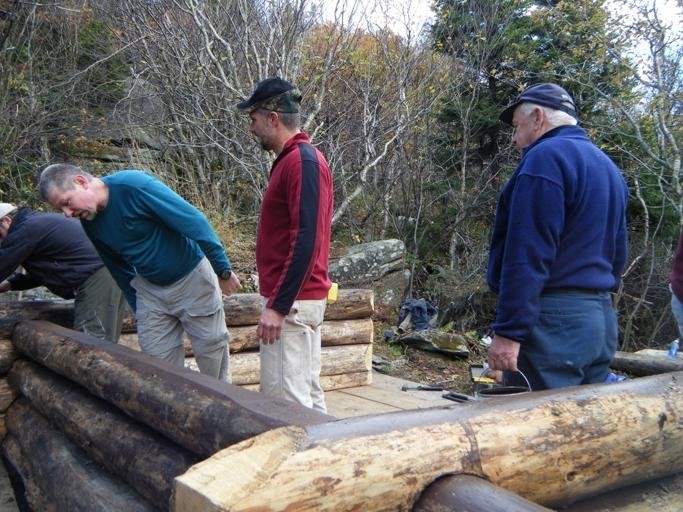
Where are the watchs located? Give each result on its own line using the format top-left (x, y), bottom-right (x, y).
top-left (217, 270), bottom-right (231, 280)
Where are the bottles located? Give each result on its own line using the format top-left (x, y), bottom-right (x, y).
top-left (667, 339), bottom-right (680, 359)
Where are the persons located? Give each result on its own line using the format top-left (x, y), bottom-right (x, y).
top-left (672, 224), bottom-right (683, 338)
top-left (237, 77), bottom-right (333, 414)
top-left (0, 203), bottom-right (126, 343)
top-left (40, 164), bottom-right (242, 383)
top-left (487, 84), bottom-right (628, 391)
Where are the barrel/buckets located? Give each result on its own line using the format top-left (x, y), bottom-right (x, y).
top-left (469, 362), bottom-right (534, 397)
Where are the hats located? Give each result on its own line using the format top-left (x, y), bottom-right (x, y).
top-left (0, 203), bottom-right (18, 219)
top-left (237, 78), bottom-right (303, 115)
top-left (498, 82), bottom-right (577, 127)
